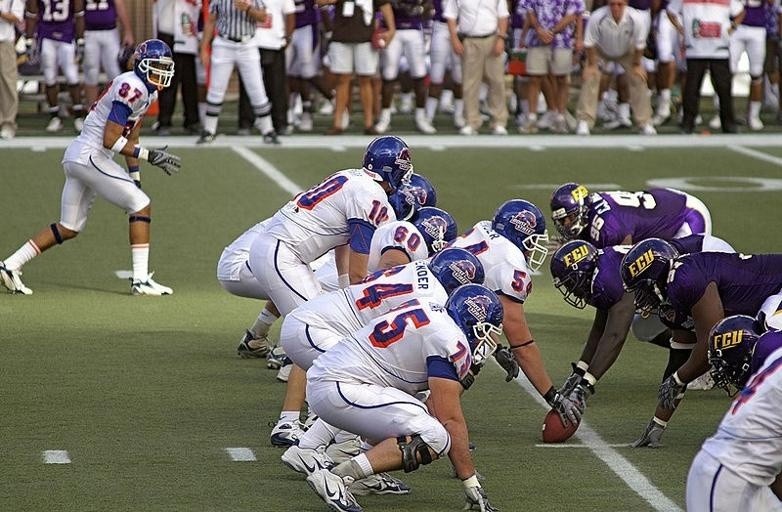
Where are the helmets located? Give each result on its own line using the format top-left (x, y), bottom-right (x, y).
top-left (133, 38), bottom-right (176, 91)
top-left (363, 136), bottom-right (503, 366)
top-left (550, 240), bottom-right (598, 309)
top-left (708, 315), bottom-right (762, 398)
top-left (619, 238), bottom-right (679, 318)
top-left (551, 183), bottom-right (592, 242)
top-left (492, 199), bottom-right (549, 272)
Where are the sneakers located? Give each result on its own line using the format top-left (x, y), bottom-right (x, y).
top-left (254, 118), bottom-right (281, 143)
top-left (268, 414), bottom-right (411, 512)
top-left (159, 122), bottom-right (214, 143)
top-left (0, 260), bottom-right (33, 295)
top-left (238, 329), bottom-right (293, 383)
top-left (129, 271), bottom-right (173, 296)
top-left (46, 116), bottom-right (85, 134)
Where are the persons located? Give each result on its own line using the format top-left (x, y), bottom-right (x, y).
top-left (0, 0), bottom-right (27, 141)
top-left (30, 0), bottom-right (87, 135)
top-left (151, 0), bottom-right (781, 145)
top-left (218, 135), bottom-right (580, 510)
top-left (0, 37), bottom-right (183, 297)
top-left (81, 0), bottom-right (135, 113)
top-left (550, 183), bottom-right (782, 511)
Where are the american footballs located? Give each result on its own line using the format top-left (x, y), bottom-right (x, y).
top-left (543, 409), bottom-right (582, 442)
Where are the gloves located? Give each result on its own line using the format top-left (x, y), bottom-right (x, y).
top-left (492, 343), bottom-right (519, 382)
top-left (148, 145), bottom-right (181, 175)
top-left (543, 362), bottom-right (595, 427)
top-left (657, 374), bottom-right (682, 410)
top-left (632, 421), bottom-right (667, 449)
top-left (461, 473), bottom-right (499, 512)
